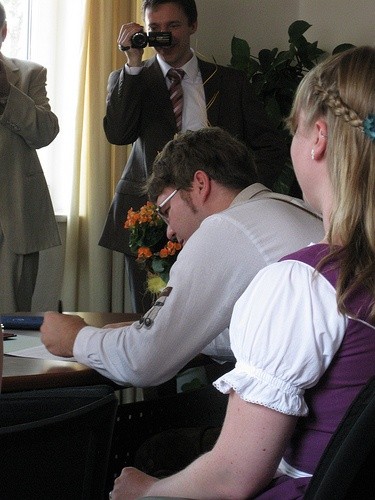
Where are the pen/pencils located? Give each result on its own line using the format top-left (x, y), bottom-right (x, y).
top-left (58, 299), bottom-right (63, 314)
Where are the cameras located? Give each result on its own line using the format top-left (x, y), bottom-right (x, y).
top-left (130, 26), bottom-right (173, 48)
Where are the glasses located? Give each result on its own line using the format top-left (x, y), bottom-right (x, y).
top-left (153, 186), bottom-right (179, 225)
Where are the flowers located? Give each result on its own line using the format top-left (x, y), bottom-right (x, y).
top-left (124, 200), bottom-right (182, 293)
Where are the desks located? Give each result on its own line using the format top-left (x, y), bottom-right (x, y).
top-left (0, 311), bottom-right (207, 500)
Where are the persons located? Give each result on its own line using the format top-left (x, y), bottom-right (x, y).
top-left (41, 124), bottom-right (329, 388)
top-left (98, 0), bottom-right (267, 313)
top-left (0, 2), bottom-right (61, 311)
top-left (111, 45), bottom-right (375, 500)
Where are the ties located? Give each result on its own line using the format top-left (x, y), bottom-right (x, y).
top-left (165, 68), bottom-right (185, 139)
